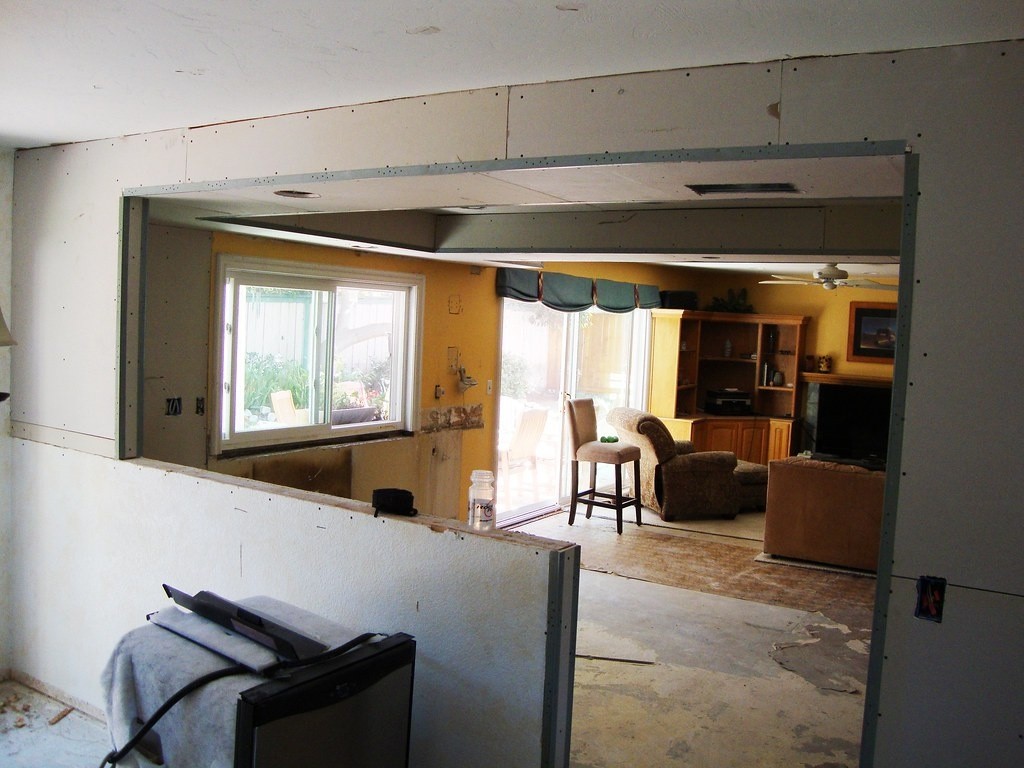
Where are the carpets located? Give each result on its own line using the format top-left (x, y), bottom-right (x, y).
top-left (518, 517), bottom-right (878, 613)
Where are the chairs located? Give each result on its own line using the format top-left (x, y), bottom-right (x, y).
top-left (564, 397), bottom-right (642, 536)
top-left (499, 408), bottom-right (549, 511)
top-left (270, 390), bottom-right (298, 424)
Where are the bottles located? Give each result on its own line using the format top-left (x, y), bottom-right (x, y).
top-left (723, 338), bottom-right (732, 357)
top-left (804, 355), bottom-right (817, 371)
top-left (468, 469), bottom-right (494, 532)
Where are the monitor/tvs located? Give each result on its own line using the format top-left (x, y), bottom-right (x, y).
top-left (814, 383), bottom-right (892, 466)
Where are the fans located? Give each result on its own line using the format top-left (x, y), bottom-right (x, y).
top-left (757, 264), bottom-right (899, 290)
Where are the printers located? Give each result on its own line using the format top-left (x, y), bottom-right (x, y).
top-left (704, 388), bottom-right (753, 416)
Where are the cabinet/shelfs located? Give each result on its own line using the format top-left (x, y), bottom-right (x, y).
top-left (647, 309), bottom-right (811, 465)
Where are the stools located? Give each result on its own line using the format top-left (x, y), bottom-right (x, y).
top-left (733, 459), bottom-right (768, 514)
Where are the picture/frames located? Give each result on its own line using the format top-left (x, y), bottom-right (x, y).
top-left (846, 301), bottom-right (898, 364)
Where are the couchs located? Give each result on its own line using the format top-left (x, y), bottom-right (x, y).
top-left (627, 415), bottom-right (739, 522)
top-left (763, 452), bottom-right (885, 573)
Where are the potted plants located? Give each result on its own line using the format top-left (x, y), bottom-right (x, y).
top-left (330, 392), bottom-right (376, 424)
